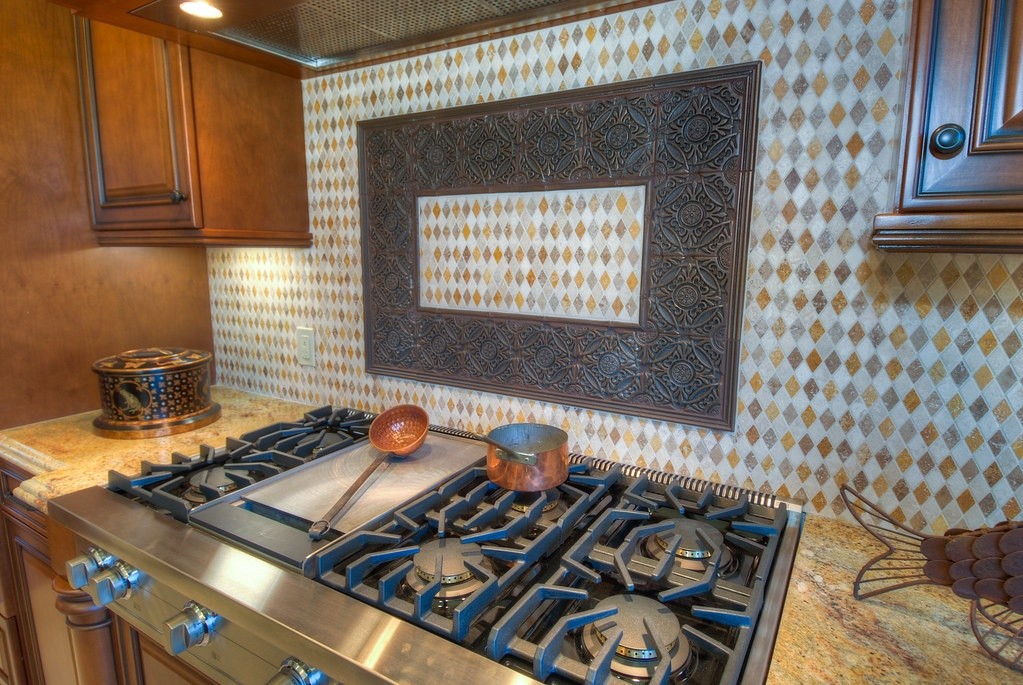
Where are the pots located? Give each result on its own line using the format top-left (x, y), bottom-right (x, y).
top-left (474, 423), bottom-right (569, 491)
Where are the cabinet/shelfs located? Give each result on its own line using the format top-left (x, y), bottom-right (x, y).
top-left (869, 0), bottom-right (1023, 254)
top-left (72, 15), bottom-right (313, 249)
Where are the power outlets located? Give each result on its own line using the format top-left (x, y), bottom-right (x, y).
top-left (296, 326), bottom-right (315, 366)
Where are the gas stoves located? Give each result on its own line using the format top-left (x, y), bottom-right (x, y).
top-left (47, 405), bottom-right (803, 685)
